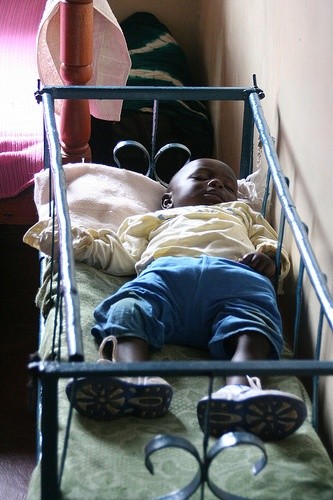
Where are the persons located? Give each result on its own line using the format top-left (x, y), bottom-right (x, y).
top-left (23, 159), bottom-right (307, 442)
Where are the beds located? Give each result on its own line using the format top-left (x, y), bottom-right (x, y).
top-left (0, 0), bottom-right (94, 228)
top-left (28, 76), bottom-right (333, 500)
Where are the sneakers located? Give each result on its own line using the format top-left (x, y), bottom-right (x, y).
top-left (65, 358), bottom-right (174, 422)
top-left (197, 375), bottom-right (307, 441)
